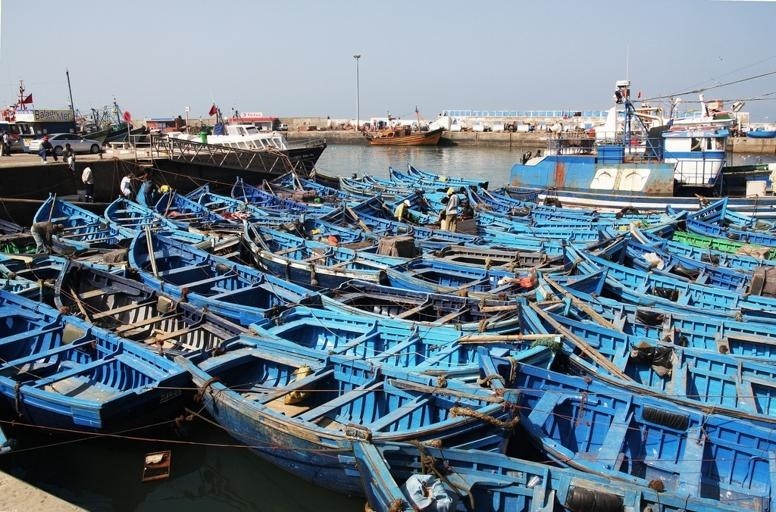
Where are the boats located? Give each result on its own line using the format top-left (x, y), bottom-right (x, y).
top-left (0, 165), bottom-right (776, 512)
top-left (747, 129), bottom-right (775, 138)
top-left (81, 123), bottom-right (151, 146)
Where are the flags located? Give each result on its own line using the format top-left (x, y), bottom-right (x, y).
top-left (209, 105), bottom-right (217, 116)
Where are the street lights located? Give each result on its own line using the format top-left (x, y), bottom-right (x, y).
top-left (352, 54), bottom-right (361, 131)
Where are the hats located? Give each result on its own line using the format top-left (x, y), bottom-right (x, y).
top-left (44, 136), bottom-right (48, 141)
top-left (404, 200), bottom-right (411, 207)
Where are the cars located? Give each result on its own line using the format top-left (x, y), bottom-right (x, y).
top-left (29, 132), bottom-right (102, 157)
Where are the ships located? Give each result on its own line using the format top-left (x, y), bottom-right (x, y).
top-left (369, 126), bottom-right (443, 146)
top-left (511, 109), bottom-right (776, 221)
top-left (163, 125), bottom-right (326, 174)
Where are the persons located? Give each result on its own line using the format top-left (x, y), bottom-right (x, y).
top-left (120, 174), bottom-right (132, 200)
top-left (62, 143), bottom-right (75, 174)
top-left (81, 163), bottom-right (94, 201)
top-left (446, 187), bottom-right (459, 232)
top-left (39, 136), bottom-right (58, 163)
top-left (0, 132), bottom-right (13, 156)
top-left (135, 172), bottom-right (151, 207)
